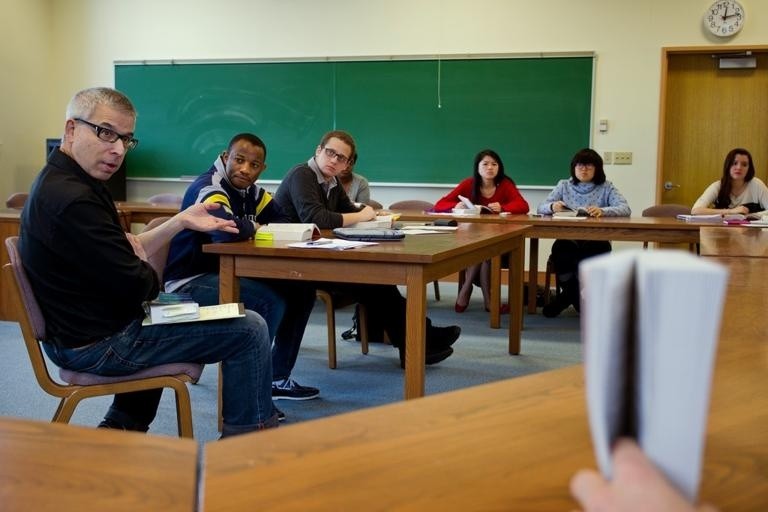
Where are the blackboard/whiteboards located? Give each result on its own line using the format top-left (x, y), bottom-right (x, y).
top-left (114, 55), bottom-right (593, 188)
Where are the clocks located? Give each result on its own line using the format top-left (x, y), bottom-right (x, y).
top-left (704, 0), bottom-right (745, 37)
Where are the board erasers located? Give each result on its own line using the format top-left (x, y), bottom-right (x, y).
top-left (182, 176), bottom-right (199, 181)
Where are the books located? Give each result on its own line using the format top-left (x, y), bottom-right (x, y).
top-left (579, 249), bottom-right (731, 501)
top-left (677, 213), bottom-right (722, 223)
top-left (723, 210), bottom-right (768, 221)
top-left (255, 223), bottom-right (321, 241)
top-left (148, 291), bottom-right (200, 325)
top-left (346, 211), bottom-right (404, 230)
top-left (551, 202), bottom-right (590, 218)
top-left (255, 240), bottom-right (310, 248)
top-left (452, 195), bottom-right (491, 216)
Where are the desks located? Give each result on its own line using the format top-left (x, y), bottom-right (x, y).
top-left (0, 202), bottom-right (768, 512)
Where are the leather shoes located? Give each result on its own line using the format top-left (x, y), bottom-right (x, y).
top-left (455, 285), bottom-right (473, 313)
top-left (484, 303), bottom-right (511, 314)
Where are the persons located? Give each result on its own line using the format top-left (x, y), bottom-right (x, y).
top-left (16, 87), bottom-right (280, 443)
top-left (537, 147), bottom-right (631, 317)
top-left (690, 148), bottom-right (767, 215)
top-left (160, 133), bottom-right (321, 402)
top-left (337, 150), bottom-right (371, 206)
top-left (272, 132), bottom-right (461, 371)
top-left (432, 150), bottom-right (529, 314)
top-left (570, 435), bottom-right (717, 512)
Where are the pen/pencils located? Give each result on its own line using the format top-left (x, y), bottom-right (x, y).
top-left (307, 240), bottom-right (333, 245)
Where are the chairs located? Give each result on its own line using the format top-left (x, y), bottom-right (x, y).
top-left (145, 194), bottom-right (182, 225)
top-left (6, 193), bottom-right (28, 208)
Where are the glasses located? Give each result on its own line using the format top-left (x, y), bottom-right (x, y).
top-left (325, 148), bottom-right (348, 163)
top-left (73, 117), bottom-right (138, 151)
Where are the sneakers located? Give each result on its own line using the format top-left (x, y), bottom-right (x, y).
top-left (399, 317), bottom-right (461, 369)
top-left (271, 378), bottom-right (320, 401)
